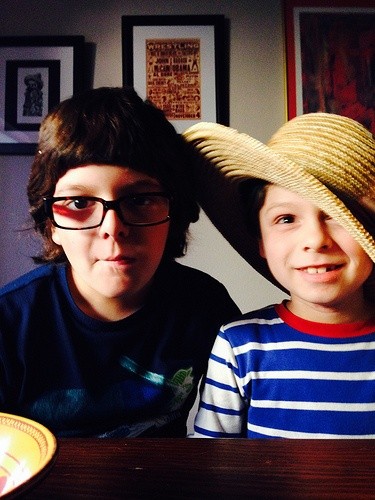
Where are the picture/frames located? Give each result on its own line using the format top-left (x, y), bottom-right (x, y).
top-left (120, 15), bottom-right (230, 138)
top-left (281, 0), bottom-right (375, 139)
top-left (0, 34), bottom-right (88, 155)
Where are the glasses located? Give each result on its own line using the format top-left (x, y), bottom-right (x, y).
top-left (43, 190), bottom-right (182, 231)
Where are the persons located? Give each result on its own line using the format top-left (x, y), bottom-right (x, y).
top-left (1, 87), bottom-right (242, 436)
top-left (180, 112), bottom-right (375, 440)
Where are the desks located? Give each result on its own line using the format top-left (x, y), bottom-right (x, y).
top-left (0, 439), bottom-right (375, 500)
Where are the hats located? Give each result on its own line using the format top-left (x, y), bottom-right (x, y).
top-left (182, 112), bottom-right (375, 297)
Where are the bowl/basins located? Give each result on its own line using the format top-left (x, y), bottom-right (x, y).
top-left (0, 413), bottom-right (59, 500)
